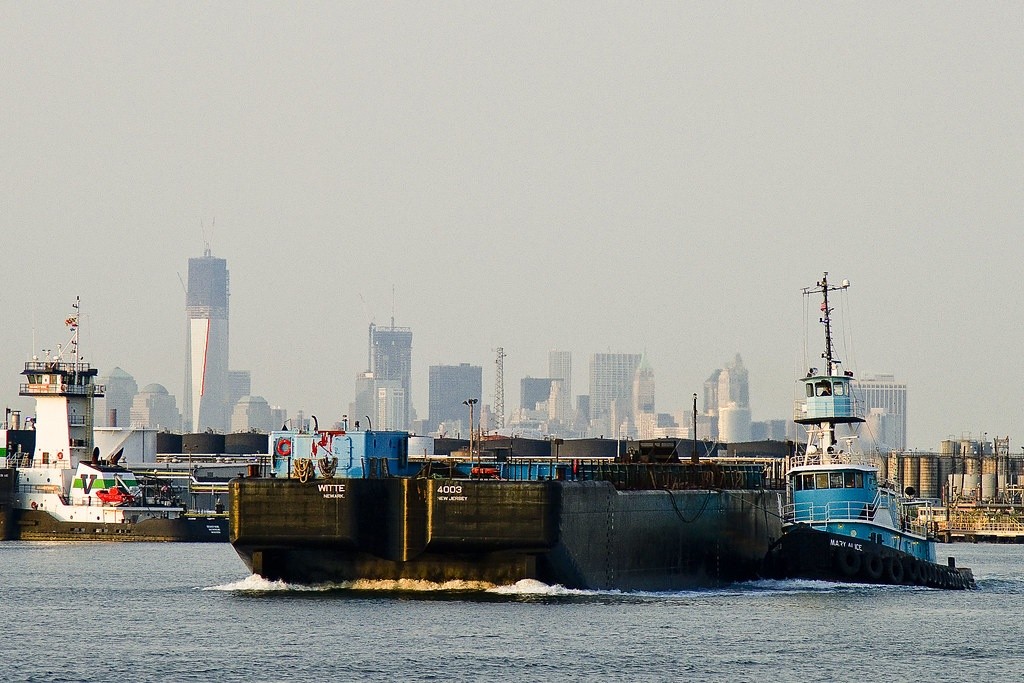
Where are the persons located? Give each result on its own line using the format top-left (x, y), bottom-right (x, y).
top-left (820, 386), bottom-right (832, 396)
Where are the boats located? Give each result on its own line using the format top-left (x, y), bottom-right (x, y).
top-left (0, 295), bottom-right (229, 544)
top-left (227, 410), bottom-right (788, 590)
top-left (95, 489), bottom-right (133, 503)
top-left (769, 270), bottom-right (975, 589)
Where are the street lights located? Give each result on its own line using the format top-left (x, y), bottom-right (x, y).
top-left (463, 398), bottom-right (478, 463)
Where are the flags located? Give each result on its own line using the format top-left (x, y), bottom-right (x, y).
top-left (65, 318), bottom-right (78, 331)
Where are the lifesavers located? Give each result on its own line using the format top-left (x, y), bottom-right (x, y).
top-left (905, 556), bottom-right (919, 582)
top-left (471, 466), bottom-right (499, 475)
top-left (886, 555), bottom-right (905, 585)
top-left (57, 452), bottom-right (63, 459)
top-left (837, 549), bottom-right (861, 574)
top-left (920, 560), bottom-right (967, 590)
top-left (31, 502), bottom-right (37, 509)
top-left (277, 440), bottom-right (293, 456)
top-left (864, 552), bottom-right (884, 580)
